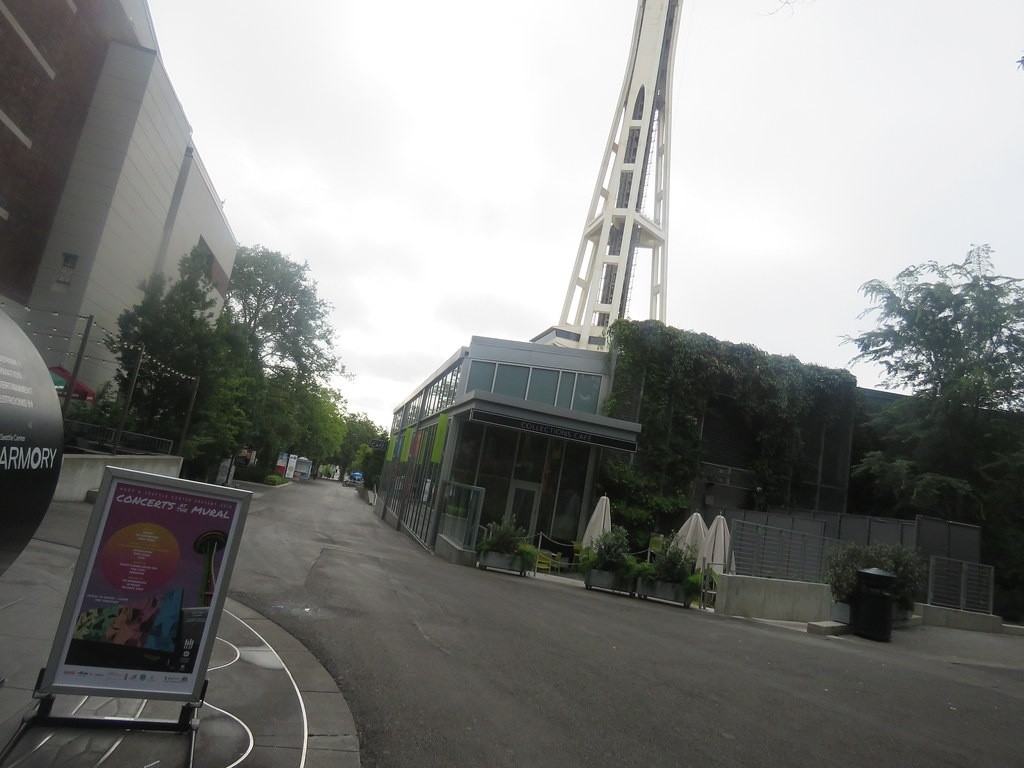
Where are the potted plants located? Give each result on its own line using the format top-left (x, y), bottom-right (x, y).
top-left (826, 542), bottom-right (927, 624)
top-left (478, 514), bottom-right (537, 577)
top-left (570, 524), bottom-right (718, 608)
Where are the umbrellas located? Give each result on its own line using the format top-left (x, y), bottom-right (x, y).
top-left (48, 362), bottom-right (95, 401)
top-left (667, 512), bottom-right (736, 576)
top-left (579, 493), bottom-right (612, 557)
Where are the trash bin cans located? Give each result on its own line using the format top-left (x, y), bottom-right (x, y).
top-left (849, 567), bottom-right (898, 641)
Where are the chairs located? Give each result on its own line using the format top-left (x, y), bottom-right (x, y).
top-left (537, 550), bottom-right (562, 575)
